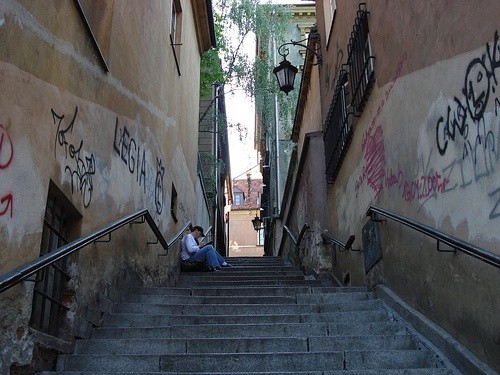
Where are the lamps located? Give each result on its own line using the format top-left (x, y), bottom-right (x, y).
top-left (272, 32), bottom-right (321, 96)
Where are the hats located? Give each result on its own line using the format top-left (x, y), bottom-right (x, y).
top-left (192, 225), bottom-right (206, 237)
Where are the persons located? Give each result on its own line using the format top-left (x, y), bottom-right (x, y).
top-left (181, 225), bottom-right (234, 272)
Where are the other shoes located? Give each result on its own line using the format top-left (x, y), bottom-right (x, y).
top-left (210, 267), bottom-right (220, 271)
top-left (221, 263), bottom-right (233, 268)
